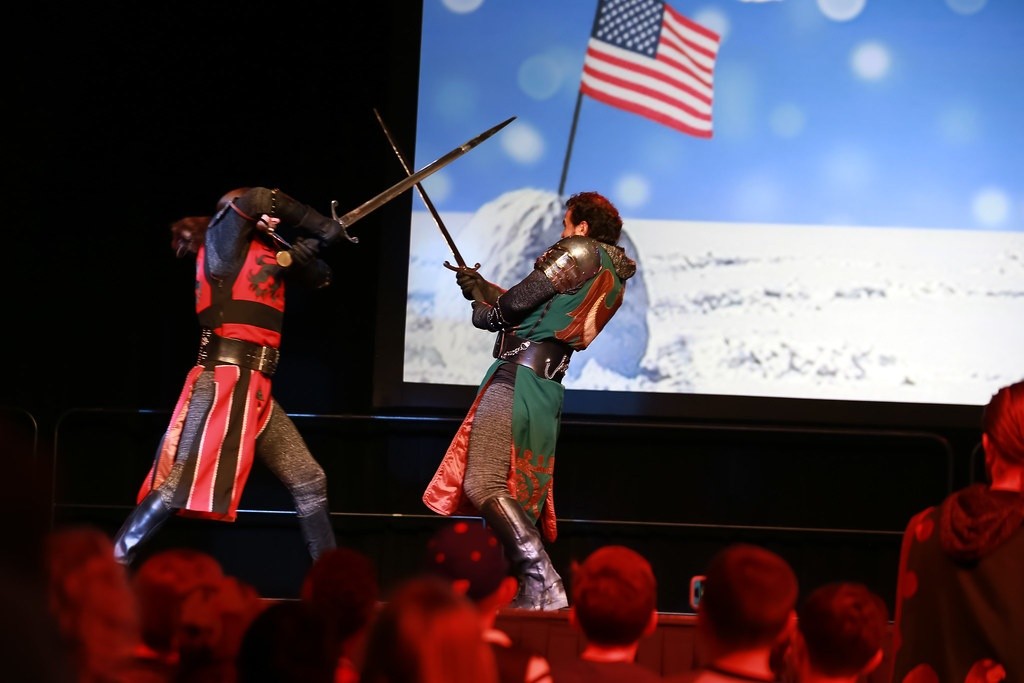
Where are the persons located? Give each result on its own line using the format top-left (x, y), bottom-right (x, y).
top-left (109, 187), bottom-right (382, 597)
top-left (777, 580), bottom-right (887, 683)
top-left (14, 519), bottom-right (553, 683)
top-left (424, 192), bottom-right (636, 612)
top-left (553, 544), bottom-right (668, 683)
top-left (888, 373), bottom-right (1023, 682)
top-left (672, 544), bottom-right (800, 683)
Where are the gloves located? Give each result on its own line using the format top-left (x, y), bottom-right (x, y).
top-left (289, 234), bottom-right (333, 293)
top-left (291, 204), bottom-right (347, 253)
top-left (456, 267), bottom-right (503, 307)
top-left (470, 301), bottom-right (499, 333)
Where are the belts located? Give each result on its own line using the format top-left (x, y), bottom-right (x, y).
top-left (491, 329), bottom-right (575, 385)
top-left (198, 332), bottom-right (281, 377)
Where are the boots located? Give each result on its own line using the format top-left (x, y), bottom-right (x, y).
top-left (479, 495), bottom-right (569, 612)
top-left (109, 489), bottom-right (176, 568)
top-left (295, 508), bottom-right (335, 566)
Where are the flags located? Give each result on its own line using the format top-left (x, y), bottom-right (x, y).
top-left (582, 2), bottom-right (722, 135)
